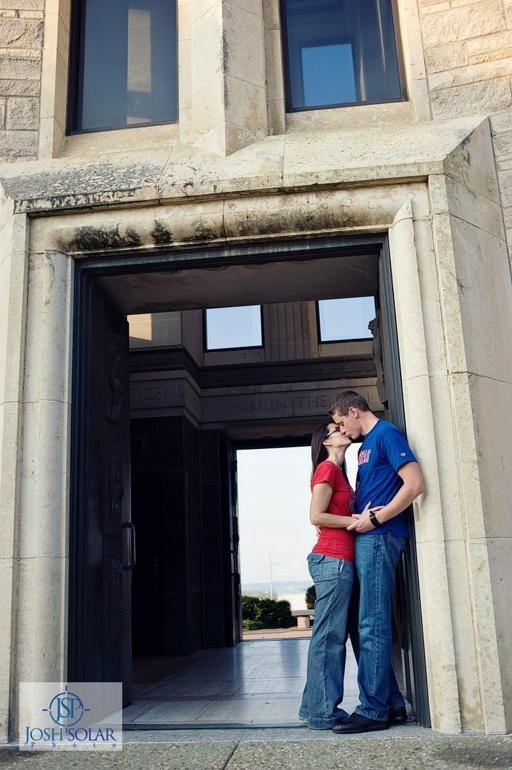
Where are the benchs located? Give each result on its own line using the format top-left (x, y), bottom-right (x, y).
top-left (291, 609), bottom-right (316, 629)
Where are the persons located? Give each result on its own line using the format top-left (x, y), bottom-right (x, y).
top-left (328, 390), bottom-right (425, 734)
top-left (298, 421), bottom-right (384, 731)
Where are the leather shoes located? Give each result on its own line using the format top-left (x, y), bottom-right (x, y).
top-left (332, 712), bottom-right (390, 734)
top-left (389, 708), bottom-right (407, 725)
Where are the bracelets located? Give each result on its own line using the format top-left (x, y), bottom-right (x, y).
top-left (369, 510), bottom-right (382, 528)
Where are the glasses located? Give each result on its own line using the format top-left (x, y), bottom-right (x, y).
top-left (327, 427), bottom-right (340, 437)
